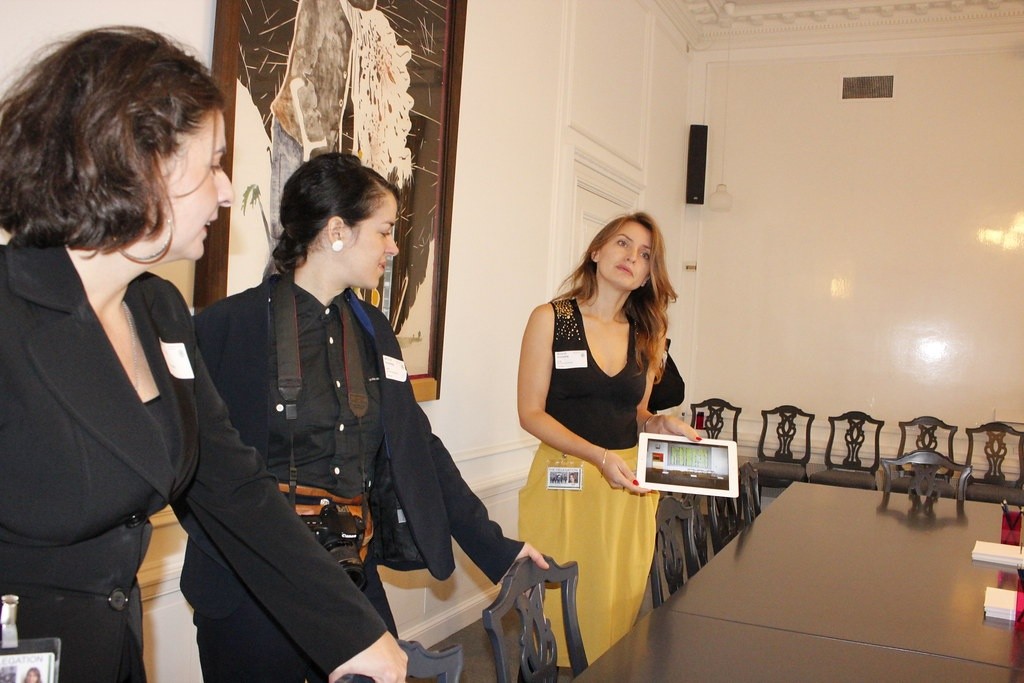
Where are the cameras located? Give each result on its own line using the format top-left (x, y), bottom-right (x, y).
top-left (299, 504), bottom-right (366, 591)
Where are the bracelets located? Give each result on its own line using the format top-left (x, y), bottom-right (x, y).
top-left (600, 449), bottom-right (608, 478)
top-left (643, 415), bottom-right (654, 432)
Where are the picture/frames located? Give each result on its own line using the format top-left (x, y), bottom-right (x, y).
top-left (193, 0), bottom-right (468, 402)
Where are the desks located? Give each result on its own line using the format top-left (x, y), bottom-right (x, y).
top-left (569, 481), bottom-right (1024, 683)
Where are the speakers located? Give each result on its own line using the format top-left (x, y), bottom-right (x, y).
top-left (686, 124), bottom-right (708, 204)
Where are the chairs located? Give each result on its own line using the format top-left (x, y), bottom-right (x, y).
top-left (334, 640), bottom-right (464, 683)
top-left (482, 553), bottom-right (588, 683)
top-left (651, 400), bottom-right (1024, 607)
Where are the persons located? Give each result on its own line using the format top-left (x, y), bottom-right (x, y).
top-left (517, 212), bottom-right (703, 667)
top-left (0, 25), bottom-right (410, 683)
top-left (569, 473), bottom-right (577, 483)
top-left (24, 667), bottom-right (41, 683)
top-left (179, 153), bottom-right (549, 683)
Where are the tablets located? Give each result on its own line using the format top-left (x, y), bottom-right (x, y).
top-left (636, 432), bottom-right (739, 498)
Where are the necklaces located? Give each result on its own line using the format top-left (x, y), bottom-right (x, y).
top-left (110, 303), bottom-right (141, 387)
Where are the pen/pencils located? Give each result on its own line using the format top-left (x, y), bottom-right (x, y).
top-left (1017, 564), bottom-right (1024, 594)
top-left (1000, 497), bottom-right (1024, 545)
